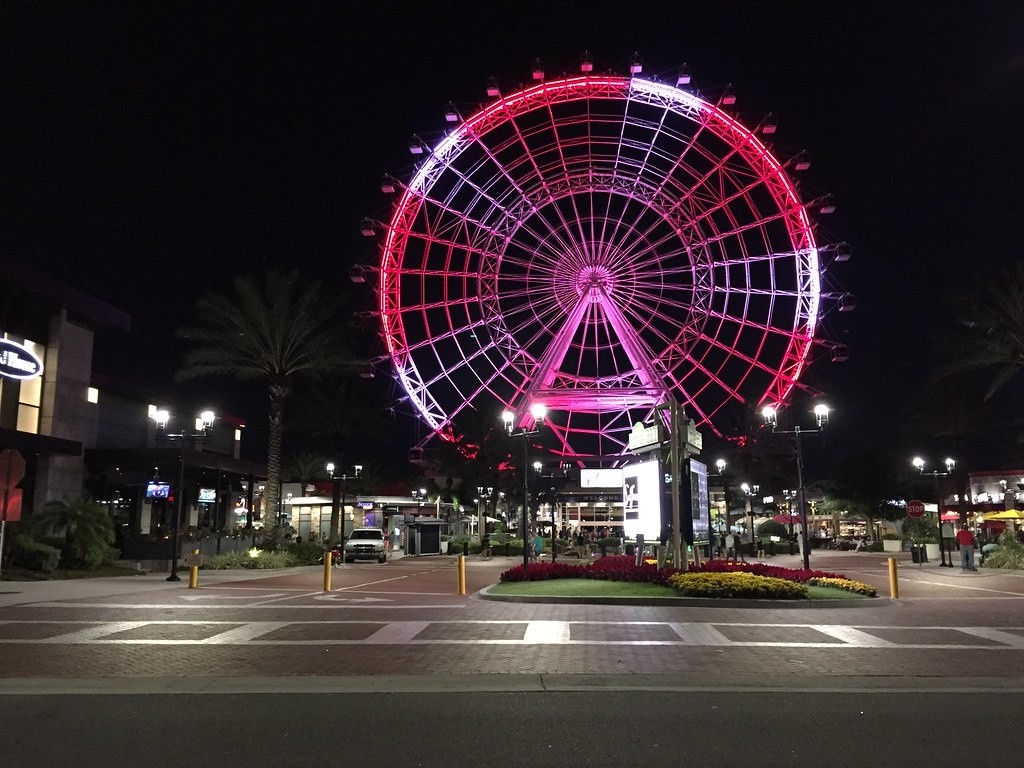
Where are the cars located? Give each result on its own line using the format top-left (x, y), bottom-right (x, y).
top-left (956, 530), bottom-right (979, 550)
top-left (343, 529), bottom-right (389, 563)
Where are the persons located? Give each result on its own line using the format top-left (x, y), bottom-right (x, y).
top-left (322, 532), bottom-right (327, 540)
top-left (797, 531), bottom-right (804, 561)
top-left (821, 528), bottom-right (829, 537)
top-left (976, 530), bottom-right (982, 542)
top-left (710, 530), bottom-right (746, 562)
top-left (387, 531), bottom-right (395, 557)
top-left (957, 525), bottom-right (976, 570)
top-left (529, 533), bottom-right (544, 564)
top-left (479, 534), bottom-right (491, 561)
top-left (538, 526), bottom-right (609, 559)
top-left (850, 529), bottom-right (855, 534)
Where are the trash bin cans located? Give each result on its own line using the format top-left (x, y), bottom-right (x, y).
top-left (910, 546), bottom-right (924, 563)
top-left (625, 544), bottom-right (635, 556)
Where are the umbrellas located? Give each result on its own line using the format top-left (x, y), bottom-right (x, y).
top-left (991, 509), bottom-right (1024, 519)
top-left (753, 517), bottom-right (769, 525)
top-left (774, 513), bottom-right (811, 527)
top-left (971, 511), bottom-right (1002, 521)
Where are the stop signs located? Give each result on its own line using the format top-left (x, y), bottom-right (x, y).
top-left (907, 500), bottom-right (924, 518)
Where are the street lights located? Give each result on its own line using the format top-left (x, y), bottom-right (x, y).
top-left (477, 486), bottom-right (493, 534)
top-left (783, 489), bottom-right (797, 554)
top-left (411, 489), bottom-right (426, 517)
top-left (534, 460), bottom-right (571, 563)
top-left (503, 404), bottom-right (546, 572)
top-left (741, 483), bottom-right (760, 557)
top-left (913, 458), bottom-right (955, 566)
top-left (154, 409), bottom-right (215, 581)
top-left (762, 404), bottom-right (828, 570)
top-left (327, 463), bottom-right (362, 564)
top-left (707, 459), bottom-right (726, 560)
